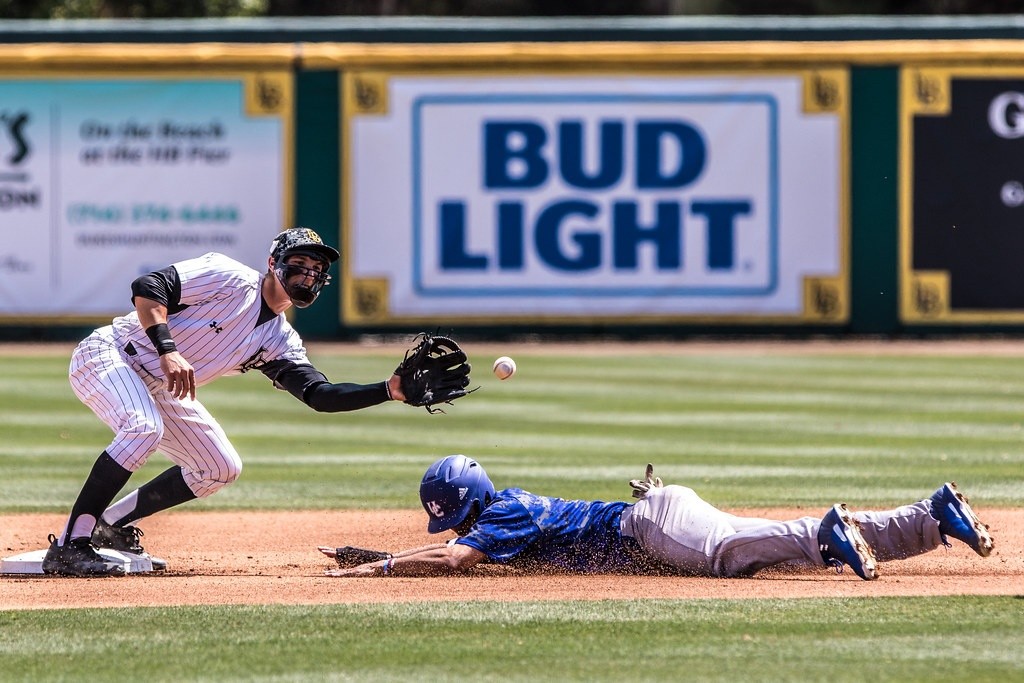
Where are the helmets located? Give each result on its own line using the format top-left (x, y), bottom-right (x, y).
top-left (270, 227), bottom-right (340, 304)
top-left (420, 455), bottom-right (495, 535)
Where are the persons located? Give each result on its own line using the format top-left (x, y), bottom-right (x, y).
top-left (43, 226), bottom-right (471, 577)
top-left (318, 453), bottom-right (996, 581)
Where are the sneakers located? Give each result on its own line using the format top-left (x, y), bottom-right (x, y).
top-left (90, 514), bottom-right (167, 570)
top-left (818, 503), bottom-right (881, 580)
top-left (930, 481), bottom-right (995, 557)
top-left (42, 533), bottom-right (126, 578)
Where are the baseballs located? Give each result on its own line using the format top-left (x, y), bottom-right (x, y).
top-left (494, 356), bottom-right (516, 380)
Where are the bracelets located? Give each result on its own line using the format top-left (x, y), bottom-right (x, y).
top-left (382, 558), bottom-right (395, 577)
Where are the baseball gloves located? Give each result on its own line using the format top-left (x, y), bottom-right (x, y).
top-left (394, 336), bottom-right (473, 408)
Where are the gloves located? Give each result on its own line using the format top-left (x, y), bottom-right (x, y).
top-left (630, 464), bottom-right (663, 499)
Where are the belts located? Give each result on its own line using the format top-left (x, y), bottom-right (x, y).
top-left (124, 342), bottom-right (155, 377)
